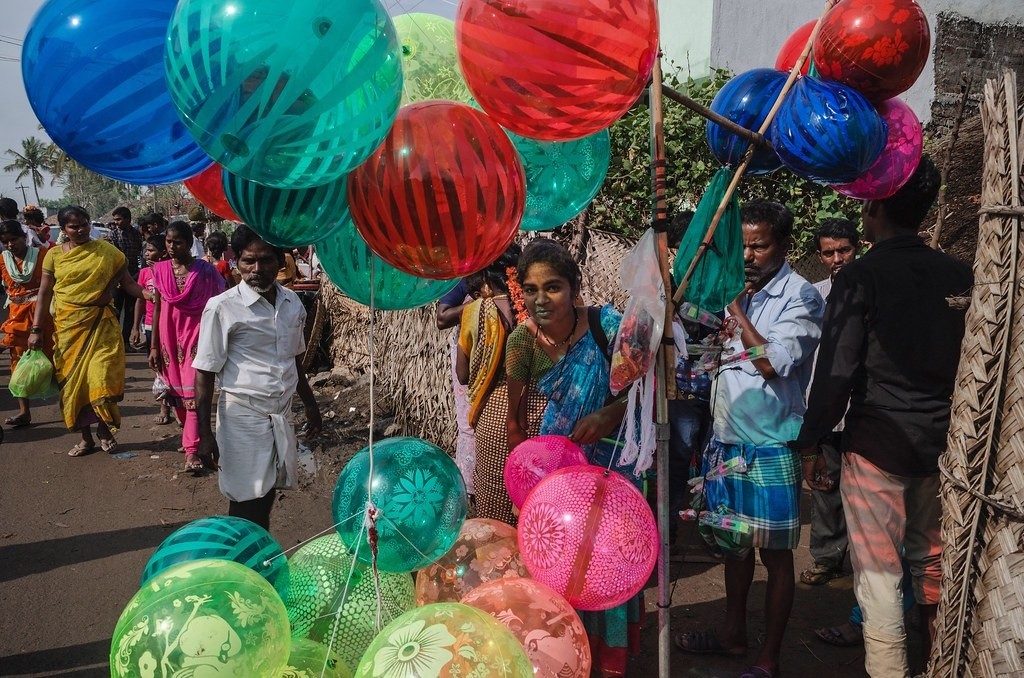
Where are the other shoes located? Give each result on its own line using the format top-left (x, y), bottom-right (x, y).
top-left (125, 344), bottom-right (137, 353)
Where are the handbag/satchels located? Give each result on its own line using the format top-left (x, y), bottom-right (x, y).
top-left (8, 347), bottom-right (59, 401)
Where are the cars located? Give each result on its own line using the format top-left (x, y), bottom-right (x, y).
top-left (48, 226), bottom-right (111, 244)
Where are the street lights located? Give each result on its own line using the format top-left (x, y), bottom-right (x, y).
top-left (15, 186), bottom-right (30, 207)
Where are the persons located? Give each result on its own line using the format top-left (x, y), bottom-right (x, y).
top-left (192, 223), bottom-right (323, 535)
top-left (788, 154), bottom-right (974, 678)
top-left (437, 196), bottom-right (860, 678)
top-left (0, 197), bottom-right (325, 473)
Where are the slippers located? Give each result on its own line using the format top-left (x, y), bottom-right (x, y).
top-left (96, 432), bottom-right (118, 453)
top-left (801, 563), bottom-right (835, 585)
top-left (155, 414), bottom-right (169, 425)
top-left (5, 416), bottom-right (30, 425)
top-left (740, 664), bottom-right (771, 678)
top-left (68, 444), bottom-right (95, 459)
top-left (674, 625), bottom-right (745, 659)
top-left (184, 461), bottom-right (204, 473)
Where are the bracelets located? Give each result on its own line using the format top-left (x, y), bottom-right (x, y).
top-left (32, 324), bottom-right (41, 327)
top-left (801, 446), bottom-right (823, 462)
top-left (150, 346), bottom-right (158, 349)
top-left (149, 294), bottom-right (156, 302)
top-left (30, 328), bottom-right (42, 334)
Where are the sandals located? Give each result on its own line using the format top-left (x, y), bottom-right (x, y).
top-left (814, 620), bottom-right (865, 645)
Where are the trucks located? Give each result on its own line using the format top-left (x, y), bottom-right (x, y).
top-left (37, 206), bottom-right (57, 219)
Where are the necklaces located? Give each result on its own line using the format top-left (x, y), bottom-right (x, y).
top-left (517, 308), bottom-right (577, 433)
top-left (539, 305), bottom-right (579, 347)
